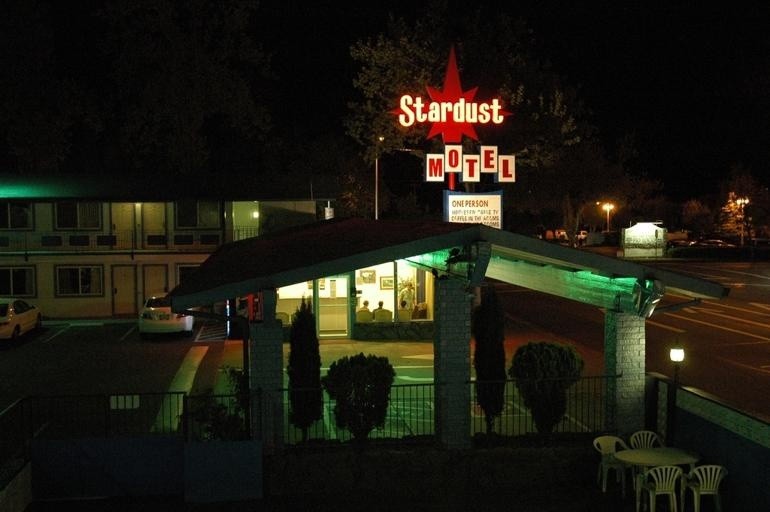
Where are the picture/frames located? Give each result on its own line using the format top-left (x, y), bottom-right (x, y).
top-left (380, 275), bottom-right (394, 290)
top-left (360, 270), bottom-right (376, 284)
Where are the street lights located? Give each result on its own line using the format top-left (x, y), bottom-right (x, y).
top-left (374, 134), bottom-right (384, 220)
top-left (602, 202), bottom-right (614, 232)
top-left (668, 333), bottom-right (686, 383)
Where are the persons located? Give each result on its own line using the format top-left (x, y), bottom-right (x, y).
top-left (358, 301), bottom-right (370, 312)
top-left (578, 231), bottom-right (584, 248)
top-left (398, 300), bottom-right (409, 311)
top-left (372, 301), bottom-right (383, 320)
top-left (557, 231), bottom-right (561, 240)
top-left (399, 282), bottom-right (417, 320)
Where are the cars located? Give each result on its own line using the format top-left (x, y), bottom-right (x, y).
top-left (0, 297), bottom-right (42, 341)
top-left (136, 293), bottom-right (194, 340)
top-left (559, 230), bottom-right (588, 243)
top-left (667, 237), bottom-right (770, 247)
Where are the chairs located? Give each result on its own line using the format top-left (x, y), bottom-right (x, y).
top-left (594, 430), bottom-right (728, 512)
top-left (356, 310), bottom-right (411, 322)
